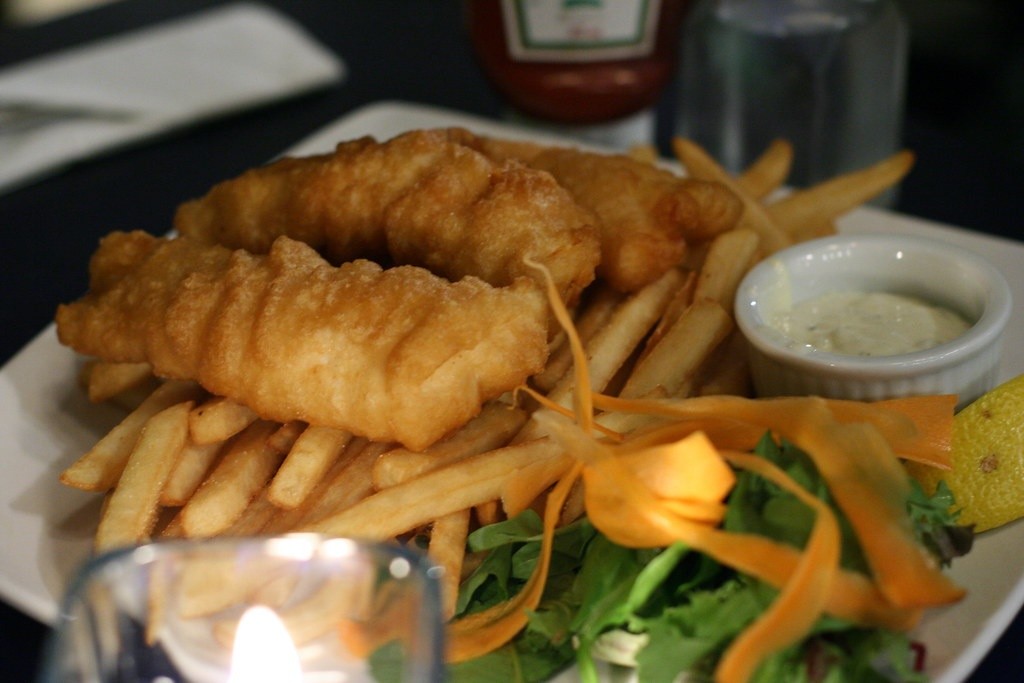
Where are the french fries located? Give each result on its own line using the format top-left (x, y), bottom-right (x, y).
top-left (62, 136), bottom-right (915, 620)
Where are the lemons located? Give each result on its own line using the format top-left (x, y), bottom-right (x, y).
top-left (902, 374), bottom-right (1024, 531)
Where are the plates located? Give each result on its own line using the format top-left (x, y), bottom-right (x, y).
top-left (0, 104), bottom-right (1024, 683)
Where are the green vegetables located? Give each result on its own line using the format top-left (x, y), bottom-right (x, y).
top-left (369, 426), bottom-right (976, 683)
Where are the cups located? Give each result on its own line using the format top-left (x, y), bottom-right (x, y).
top-left (667, 0), bottom-right (911, 217)
top-left (731, 229), bottom-right (1016, 415)
top-left (24, 528), bottom-right (445, 683)
